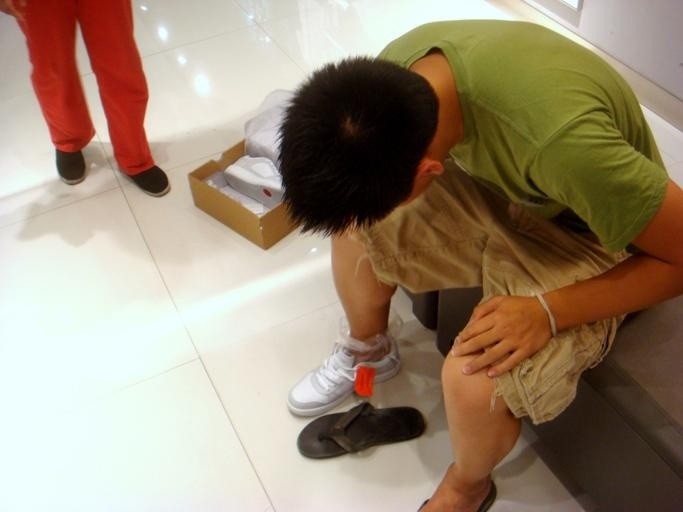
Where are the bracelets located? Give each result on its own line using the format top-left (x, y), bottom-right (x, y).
top-left (530, 290), bottom-right (559, 337)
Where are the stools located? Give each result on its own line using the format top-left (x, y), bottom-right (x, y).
top-left (401, 265), bottom-right (683, 512)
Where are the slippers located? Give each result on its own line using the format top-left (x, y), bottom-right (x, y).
top-left (297, 401), bottom-right (425, 458)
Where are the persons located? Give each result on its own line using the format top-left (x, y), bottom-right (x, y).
top-left (0, 0), bottom-right (172, 197)
top-left (277, 18), bottom-right (683, 512)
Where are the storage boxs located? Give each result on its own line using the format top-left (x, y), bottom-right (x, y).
top-left (188, 137), bottom-right (306, 250)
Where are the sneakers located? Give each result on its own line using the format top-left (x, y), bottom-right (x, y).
top-left (286, 330), bottom-right (401, 417)
top-left (128, 165), bottom-right (170, 196)
top-left (56, 147), bottom-right (85, 184)
top-left (223, 155), bottom-right (287, 211)
